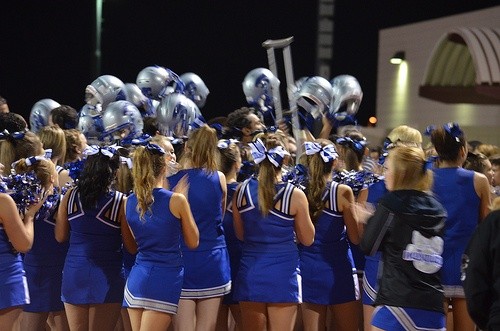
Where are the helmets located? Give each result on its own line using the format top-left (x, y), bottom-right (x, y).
top-left (296, 78), bottom-right (333, 120)
top-left (103, 101), bottom-right (144, 137)
top-left (156, 93), bottom-right (196, 138)
top-left (124, 84), bottom-right (148, 108)
top-left (136, 66), bottom-right (172, 99)
top-left (30, 98), bottom-right (61, 128)
top-left (328, 75), bottom-right (362, 116)
top-left (180, 72), bottom-right (211, 108)
top-left (77, 117), bottom-right (96, 136)
top-left (242, 66), bottom-right (280, 104)
top-left (86, 75), bottom-right (124, 110)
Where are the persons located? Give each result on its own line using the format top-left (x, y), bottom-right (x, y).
top-left (231, 133), bottom-right (314, 331)
top-left (0, 98), bottom-right (500, 331)
top-left (119, 142), bottom-right (199, 331)
